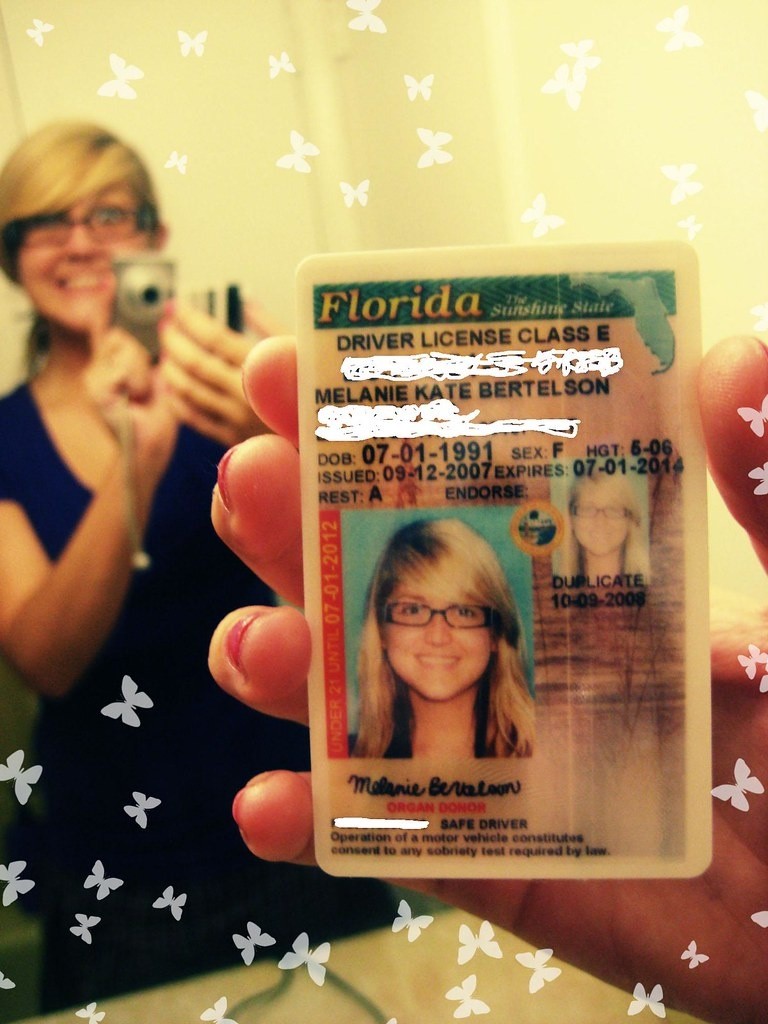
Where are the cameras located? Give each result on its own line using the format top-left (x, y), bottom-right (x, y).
top-left (111, 259), bottom-right (176, 366)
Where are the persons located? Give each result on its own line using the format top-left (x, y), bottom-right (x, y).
top-left (561, 470), bottom-right (648, 581)
top-left (204, 338), bottom-right (768, 1023)
top-left (0, 122), bottom-right (401, 1015)
top-left (348, 515), bottom-right (535, 761)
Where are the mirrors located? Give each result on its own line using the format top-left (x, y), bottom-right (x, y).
top-left (0, 0), bottom-right (768, 1024)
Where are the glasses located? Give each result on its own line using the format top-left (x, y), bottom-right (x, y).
top-left (15, 206), bottom-right (158, 247)
top-left (570, 505), bottom-right (633, 519)
top-left (376, 601), bottom-right (501, 629)
